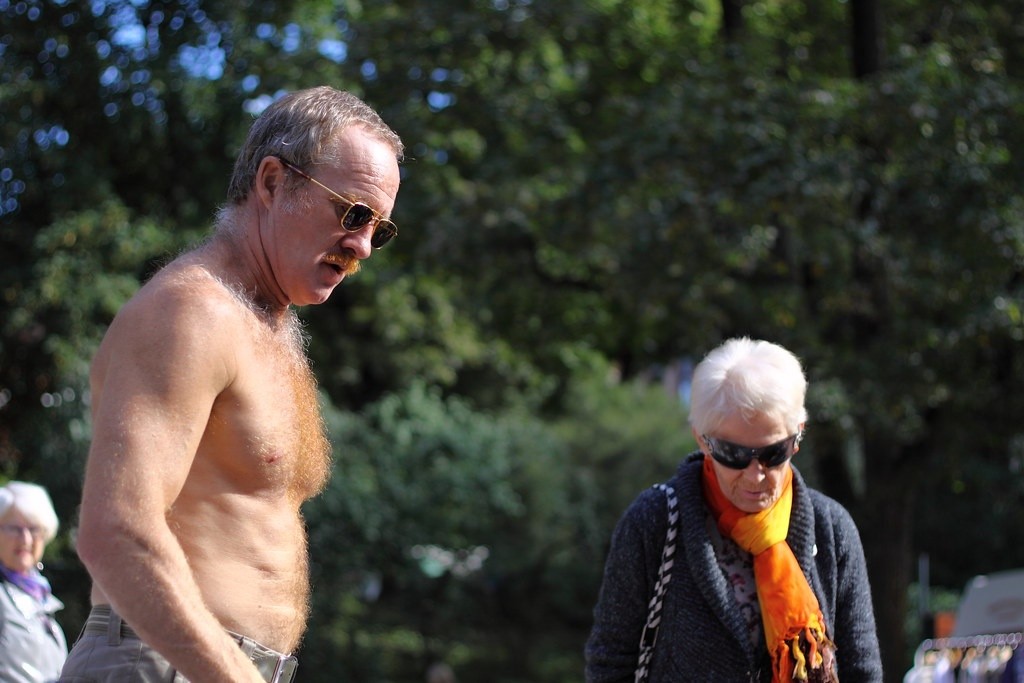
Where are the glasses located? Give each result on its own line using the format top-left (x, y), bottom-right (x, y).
top-left (0, 523), bottom-right (45, 539)
top-left (701, 428), bottom-right (799, 470)
top-left (280, 159), bottom-right (400, 250)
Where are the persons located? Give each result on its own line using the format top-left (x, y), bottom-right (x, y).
top-left (0, 481), bottom-right (68, 683)
top-left (60, 84), bottom-right (402, 683)
top-left (582, 338), bottom-right (883, 682)
